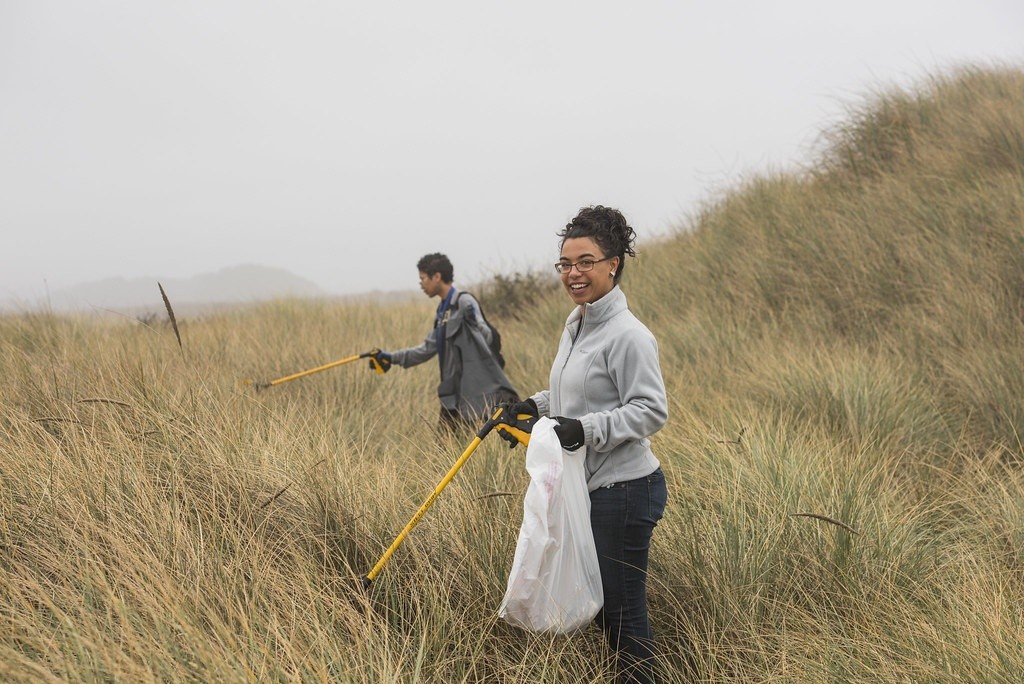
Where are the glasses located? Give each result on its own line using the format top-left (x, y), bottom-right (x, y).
top-left (555, 258), bottom-right (610, 274)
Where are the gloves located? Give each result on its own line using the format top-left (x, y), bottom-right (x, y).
top-left (549, 416), bottom-right (584, 451)
top-left (497, 402), bottom-right (538, 449)
top-left (369, 352), bottom-right (391, 368)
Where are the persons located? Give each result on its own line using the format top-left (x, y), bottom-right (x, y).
top-left (496, 206), bottom-right (667, 683)
top-left (369, 252), bottom-right (493, 430)
